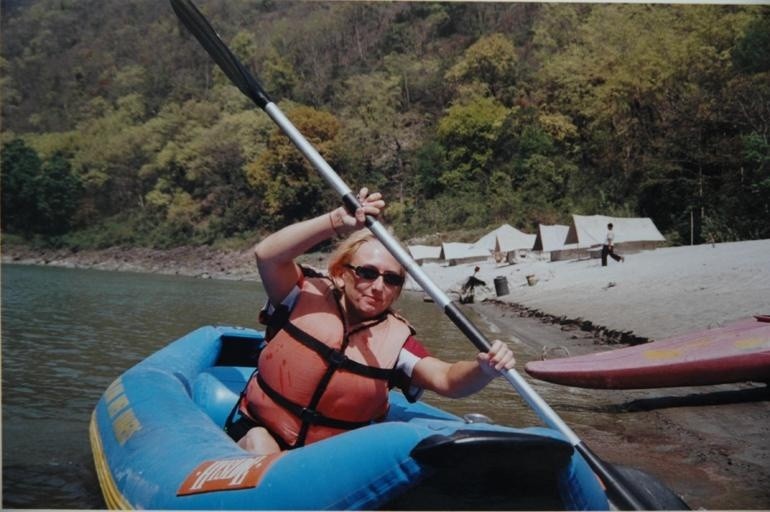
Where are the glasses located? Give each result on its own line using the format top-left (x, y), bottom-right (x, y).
top-left (342, 264), bottom-right (404, 286)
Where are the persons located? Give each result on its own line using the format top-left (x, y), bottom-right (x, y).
top-left (223, 188), bottom-right (516, 458)
top-left (598, 222), bottom-right (625, 267)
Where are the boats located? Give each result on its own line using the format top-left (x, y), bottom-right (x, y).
top-left (517, 304), bottom-right (769, 395)
top-left (86, 317), bottom-right (609, 512)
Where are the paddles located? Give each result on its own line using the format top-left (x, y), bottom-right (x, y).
top-left (170, 0), bottom-right (692, 511)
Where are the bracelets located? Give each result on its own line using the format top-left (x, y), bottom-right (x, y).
top-left (328, 209), bottom-right (351, 235)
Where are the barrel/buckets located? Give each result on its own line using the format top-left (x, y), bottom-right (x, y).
top-left (526, 273), bottom-right (535, 287)
top-left (493, 276), bottom-right (509, 296)
